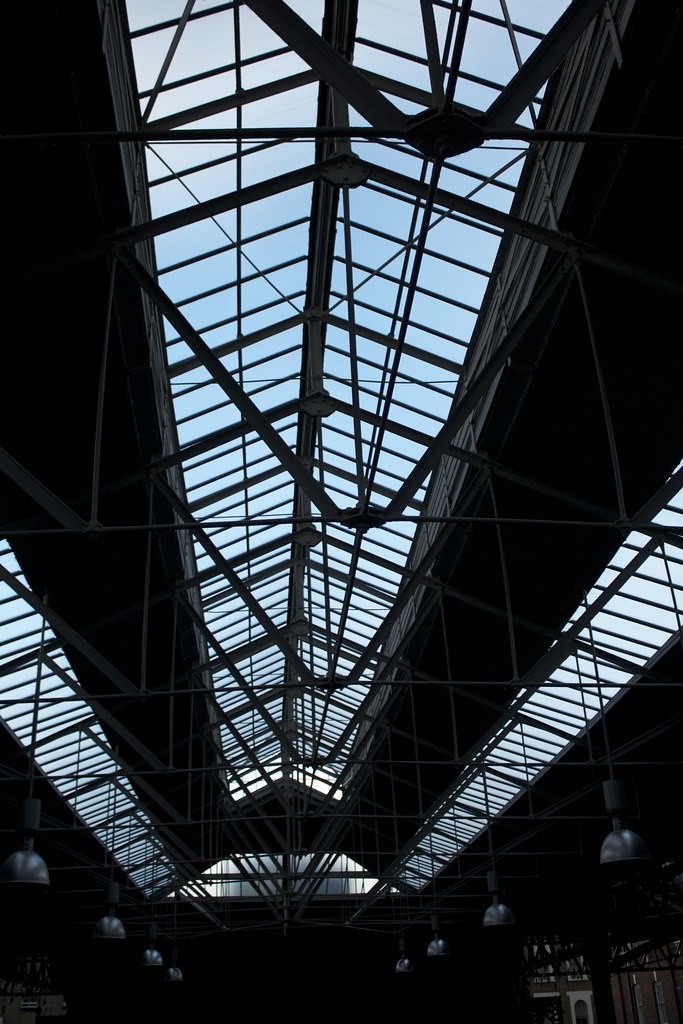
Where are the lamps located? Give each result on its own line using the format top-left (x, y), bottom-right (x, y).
top-left (396, 859), bottom-right (417, 972)
top-left (575, 580), bottom-right (652, 866)
top-left (1, 595), bottom-right (51, 884)
top-left (424, 827), bottom-right (451, 957)
top-left (165, 885), bottom-right (184, 980)
top-left (91, 743), bottom-right (127, 939)
top-left (470, 738), bottom-right (516, 927)
top-left (140, 826), bottom-right (162, 965)
